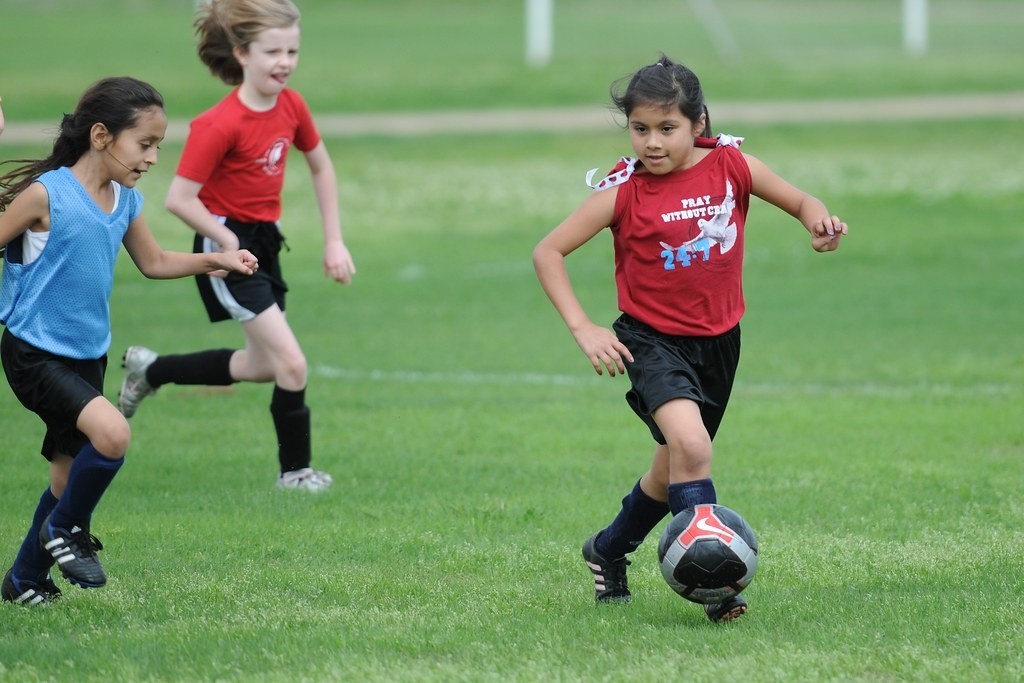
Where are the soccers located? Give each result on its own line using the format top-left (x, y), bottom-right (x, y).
top-left (657, 504), bottom-right (758, 603)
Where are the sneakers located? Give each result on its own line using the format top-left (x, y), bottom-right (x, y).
top-left (278, 467), bottom-right (333, 491)
top-left (582, 528), bottom-right (633, 608)
top-left (37, 515), bottom-right (108, 590)
top-left (116, 345), bottom-right (161, 418)
top-left (1, 568), bottom-right (62, 614)
top-left (701, 594), bottom-right (748, 622)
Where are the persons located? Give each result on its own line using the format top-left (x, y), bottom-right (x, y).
top-left (0, 77), bottom-right (258, 608)
top-left (534, 56), bottom-right (847, 626)
top-left (117, 0), bottom-right (355, 489)
